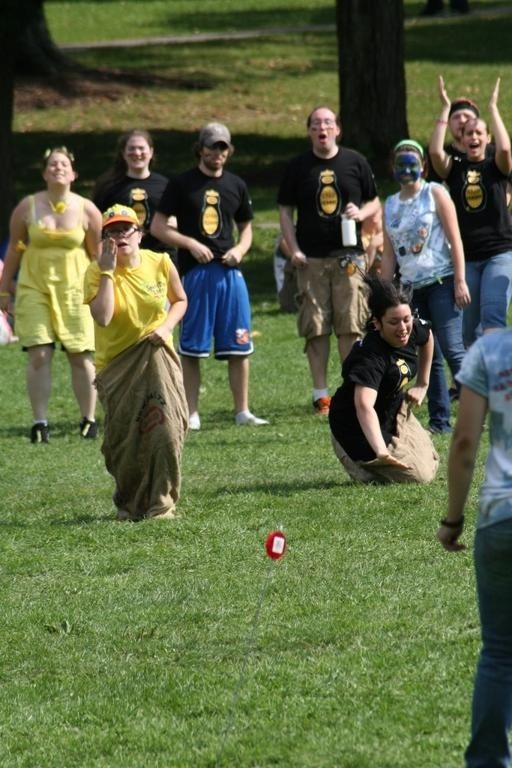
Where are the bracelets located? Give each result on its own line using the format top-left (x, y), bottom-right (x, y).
top-left (0, 290), bottom-right (11, 299)
top-left (436, 120), bottom-right (448, 125)
top-left (99, 270), bottom-right (114, 281)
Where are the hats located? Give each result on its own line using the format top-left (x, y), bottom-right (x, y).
top-left (102, 204), bottom-right (139, 230)
top-left (200, 122), bottom-right (231, 150)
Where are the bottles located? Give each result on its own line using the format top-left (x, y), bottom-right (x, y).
top-left (342, 214), bottom-right (357, 246)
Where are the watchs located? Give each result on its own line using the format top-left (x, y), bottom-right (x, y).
top-left (440, 517), bottom-right (465, 529)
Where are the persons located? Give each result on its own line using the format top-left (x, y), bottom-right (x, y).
top-left (273, 233), bottom-right (301, 313)
top-left (275, 106), bottom-right (378, 414)
top-left (0, 147), bottom-right (102, 443)
top-left (437, 326), bottom-right (512, 767)
top-left (328, 286), bottom-right (441, 487)
top-left (93, 128), bottom-right (177, 255)
top-left (427, 96), bottom-right (495, 185)
top-left (83, 203), bottom-right (187, 524)
top-left (380, 138), bottom-right (471, 436)
top-left (151, 123), bottom-right (269, 430)
top-left (429, 74), bottom-right (511, 344)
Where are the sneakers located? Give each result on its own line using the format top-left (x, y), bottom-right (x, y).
top-left (189, 412), bottom-right (201, 429)
top-left (312, 393), bottom-right (331, 414)
top-left (80, 419), bottom-right (97, 439)
top-left (236, 412), bottom-right (269, 428)
top-left (31, 424), bottom-right (48, 443)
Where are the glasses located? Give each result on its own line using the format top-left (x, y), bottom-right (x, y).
top-left (102, 226), bottom-right (139, 241)
